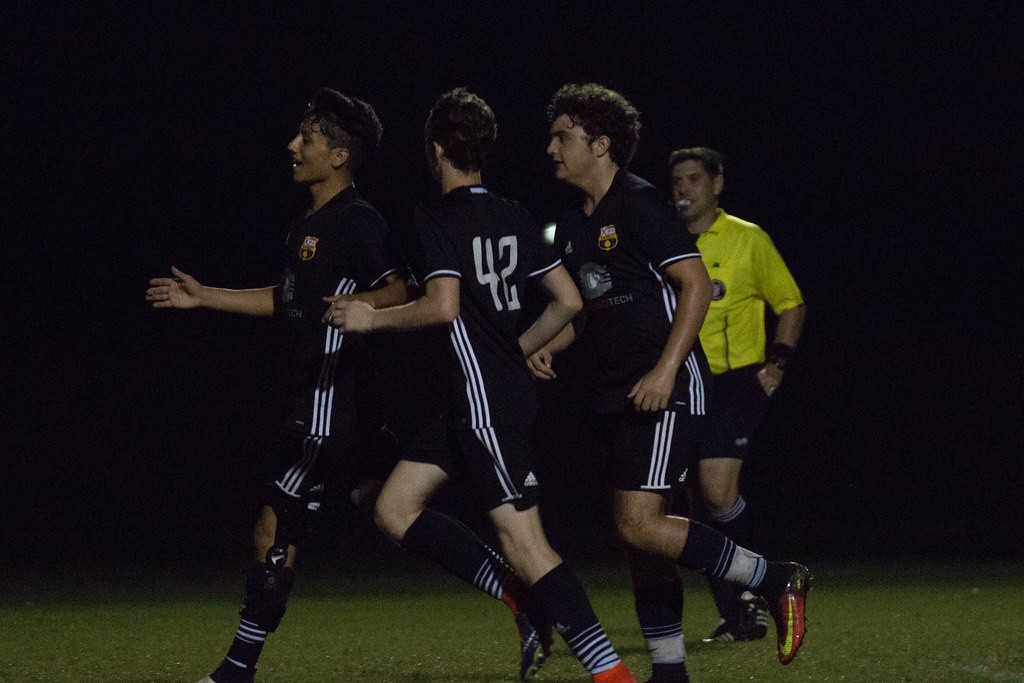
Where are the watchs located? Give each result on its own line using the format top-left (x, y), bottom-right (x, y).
top-left (769, 355), bottom-right (789, 373)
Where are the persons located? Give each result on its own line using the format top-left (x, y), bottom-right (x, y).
top-left (141, 83), bottom-right (811, 682)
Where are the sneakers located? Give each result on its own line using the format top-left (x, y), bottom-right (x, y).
top-left (757, 561), bottom-right (814, 665)
top-left (515, 589), bottom-right (555, 681)
top-left (734, 594), bottom-right (769, 643)
top-left (701, 618), bottom-right (734, 644)
